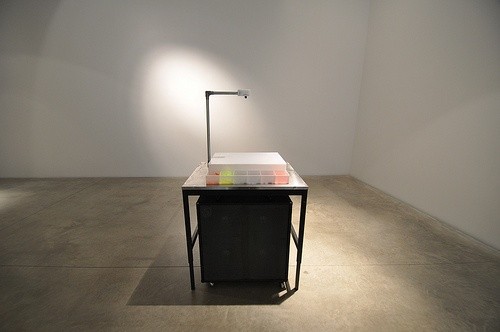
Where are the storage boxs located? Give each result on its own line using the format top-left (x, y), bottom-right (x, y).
top-left (196, 195), bottom-right (294, 289)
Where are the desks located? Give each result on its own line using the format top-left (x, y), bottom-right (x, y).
top-left (182, 162), bottom-right (309, 291)
